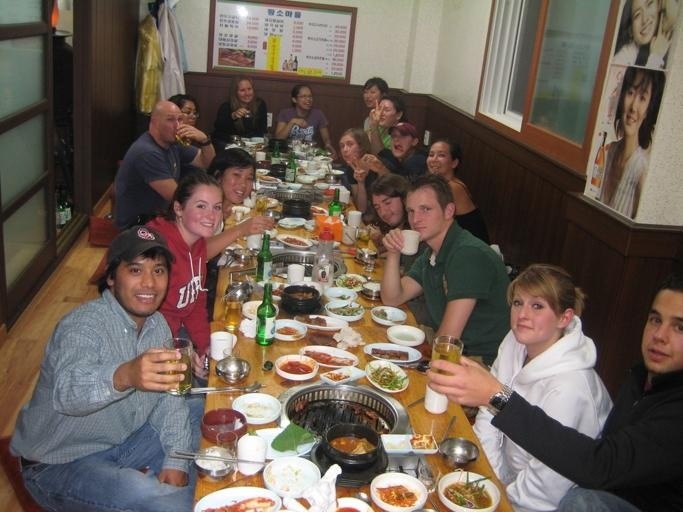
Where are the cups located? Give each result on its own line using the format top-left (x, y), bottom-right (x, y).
top-left (174, 128), bottom-right (192, 147)
top-left (207, 332), bottom-right (238, 361)
top-left (424, 384), bottom-right (448, 414)
top-left (429, 335), bottom-right (464, 380)
top-left (160, 337), bottom-right (194, 398)
top-left (216, 430), bottom-right (238, 451)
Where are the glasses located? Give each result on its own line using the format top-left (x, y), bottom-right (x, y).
top-left (181, 111), bottom-right (199, 118)
top-left (294, 95), bottom-right (313, 100)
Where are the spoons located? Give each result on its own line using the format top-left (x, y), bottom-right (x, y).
top-left (191, 382), bottom-right (262, 395)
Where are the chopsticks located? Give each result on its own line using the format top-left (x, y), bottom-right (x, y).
top-left (166, 448), bottom-right (264, 466)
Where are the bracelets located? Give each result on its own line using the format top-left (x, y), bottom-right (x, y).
top-left (198, 135), bottom-right (213, 146)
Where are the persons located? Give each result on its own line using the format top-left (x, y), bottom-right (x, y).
top-left (142, 169), bottom-right (223, 397)
top-left (168, 93), bottom-right (199, 145)
top-left (425, 260), bottom-right (683, 512)
top-left (112, 99), bottom-right (216, 232)
top-left (8, 223), bottom-right (204, 512)
top-left (597, 67), bottom-right (667, 221)
top-left (339, 77), bottom-right (511, 365)
top-left (612, 0), bottom-right (674, 72)
top-left (203, 147), bottom-right (275, 264)
top-left (471, 262), bottom-right (611, 512)
top-left (211, 73), bottom-right (267, 153)
top-left (274, 83), bottom-right (337, 160)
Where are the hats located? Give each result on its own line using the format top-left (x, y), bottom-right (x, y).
top-left (106, 225), bottom-right (177, 266)
top-left (388, 121), bottom-right (421, 139)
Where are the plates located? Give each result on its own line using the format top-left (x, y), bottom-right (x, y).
top-left (380, 432), bottom-right (439, 456)
top-left (237, 426), bottom-right (315, 460)
top-left (308, 440), bottom-right (388, 489)
top-left (313, 498), bottom-right (372, 512)
top-left (227, 137), bottom-right (427, 396)
top-left (192, 486), bottom-right (282, 511)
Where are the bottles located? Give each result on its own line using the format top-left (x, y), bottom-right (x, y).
top-left (54, 189), bottom-right (73, 228)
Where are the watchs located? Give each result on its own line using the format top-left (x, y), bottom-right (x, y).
top-left (487, 383), bottom-right (512, 419)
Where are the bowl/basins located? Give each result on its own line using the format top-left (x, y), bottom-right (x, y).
top-left (322, 423), bottom-right (385, 470)
top-left (215, 357), bottom-right (251, 386)
top-left (366, 470), bottom-right (501, 511)
top-left (231, 393), bottom-right (281, 426)
top-left (191, 450), bottom-right (235, 482)
top-left (262, 458), bottom-right (322, 498)
top-left (438, 437), bottom-right (481, 467)
top-left (200, 409), bottom-right (245, 442)
